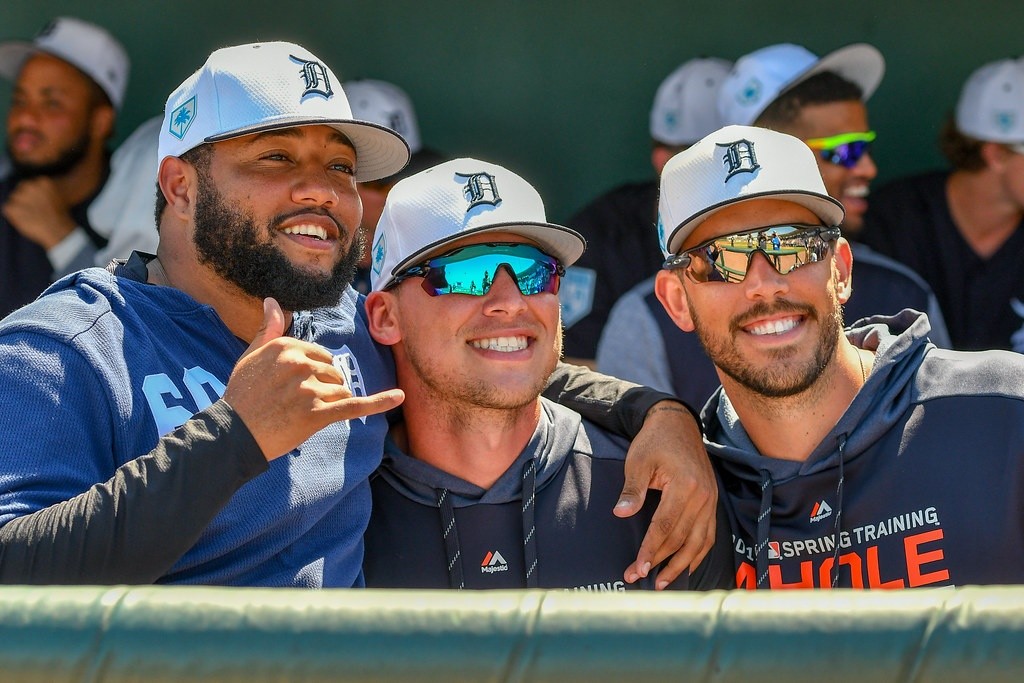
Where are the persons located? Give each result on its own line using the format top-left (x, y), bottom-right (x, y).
top-left (363, 150), bottom-right (737, 591)
top-left (559, 41), bottom-right (1024, 415)
top-left (652, 122), bottom-right (1024, 589)
top-left (1, 10), bottom-right (431, 316)
top-left (0, 38), bottom-right (720, 591)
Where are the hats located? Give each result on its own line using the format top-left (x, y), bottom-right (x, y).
top-left (157, 41), bottom-right (413, 182)
top-left (656, 122), bottom-right (848, 256)
top-left (649, 58), bottom-right (728, 144)
top-left (958, 57), bottom-right (1024, 143)
top-left (3, 17), bottom-right (127, 108)
top-left (715, 40), bottom-right (885, 127)
top-left (341, 75), bottom-right (423, 152)
top-left (366, 153), bottom-right (585, 291)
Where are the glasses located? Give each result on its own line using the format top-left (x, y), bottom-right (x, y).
top-left (386, 245), bottom-right (567, 299)
top-left (806, 133), bottom-right (881, 167)
top-left (662, 223), bottom-right (842, 284)
